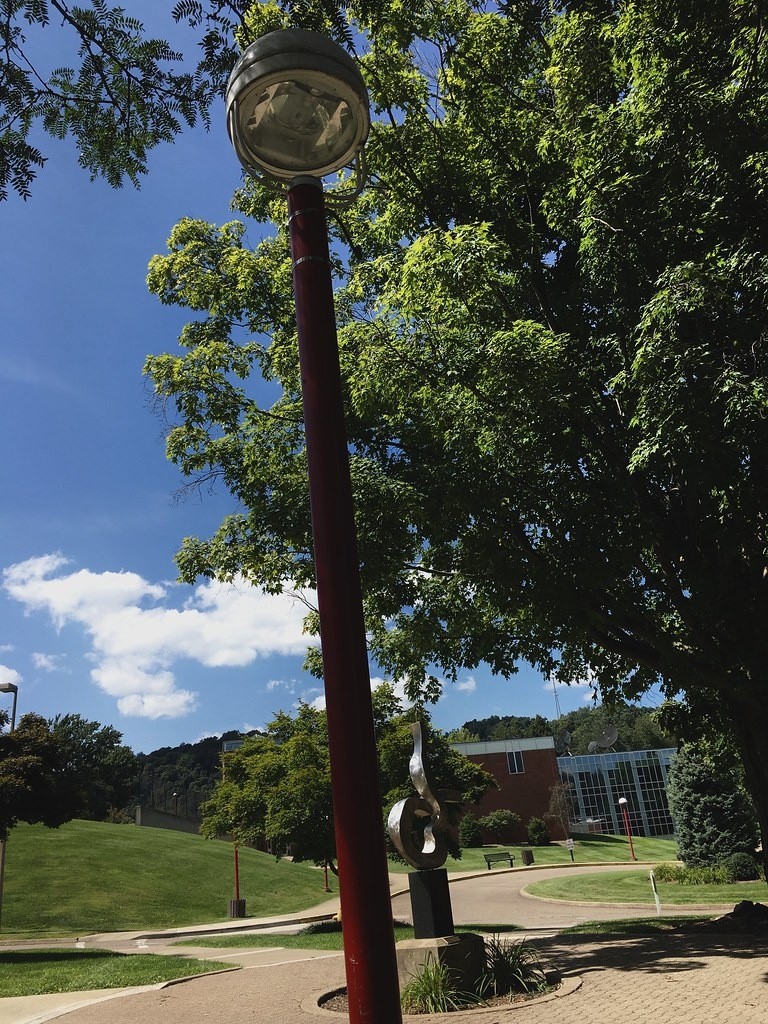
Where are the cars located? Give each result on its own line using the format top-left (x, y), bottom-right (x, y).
top-left (571, 817), bottom-right (607, 827)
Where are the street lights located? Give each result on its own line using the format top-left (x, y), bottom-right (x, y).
top-left (224, 29), bottom-right (404, 1024)
top-left (0, 682), bottom-right (18, 912)
top-left (173, 793), bottom-right (178, 815)
top-left (619, 799), bottom-right (637, 862)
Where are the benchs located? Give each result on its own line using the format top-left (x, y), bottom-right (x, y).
top-left (483, 851), bottom-right (515, 869)
